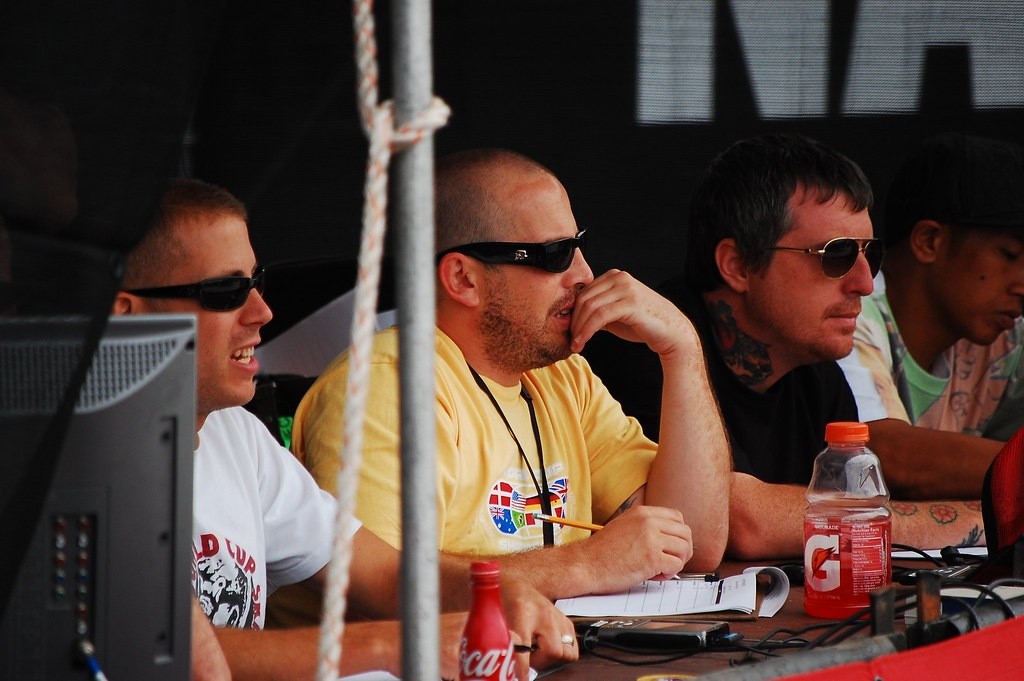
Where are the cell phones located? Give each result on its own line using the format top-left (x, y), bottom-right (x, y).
top-left (597, 619), bottom-right (730, 649)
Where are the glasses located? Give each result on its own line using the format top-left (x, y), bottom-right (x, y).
top-left (744, 237), bottom-right (884, 279)
top-left (435, 227), bottom-right (587, 272)
top-left (128, 265), bottom-right (266, 311)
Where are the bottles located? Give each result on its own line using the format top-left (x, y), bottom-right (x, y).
top-left (802, 421), bottom-right (895, 622)
top-left (457, 560), bottom-right (518, 681)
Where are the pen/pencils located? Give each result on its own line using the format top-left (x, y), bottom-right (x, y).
top-left (514, 644), bottom-right (535, 653)
top-left (676, 572), bottom-right (720, 582)
top-left (532, 513), bottom-right (605, 531)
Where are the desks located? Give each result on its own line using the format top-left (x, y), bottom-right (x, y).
top-left (539, 560), bottom-right (1024, 681)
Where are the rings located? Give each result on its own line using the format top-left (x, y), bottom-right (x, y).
top-left (561, 635), bottom-right (573, 645)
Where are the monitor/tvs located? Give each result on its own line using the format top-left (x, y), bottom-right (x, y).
top-left (0, 312), bottom-right (198, 681)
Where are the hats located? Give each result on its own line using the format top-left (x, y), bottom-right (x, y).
top-left (884, 131), bottom-right (1024, 226)
top-left (963, 425), bottom-right (1024, 582)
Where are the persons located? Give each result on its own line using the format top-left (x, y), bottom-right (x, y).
top-left (617, 132), bottom-right (987, 559)
top-left (290, 147), bottom-right (731, 599)
top-left (54, 172), bottom-right (579, 681)
top-left (836, 138), bottom-right (1024, 497)
